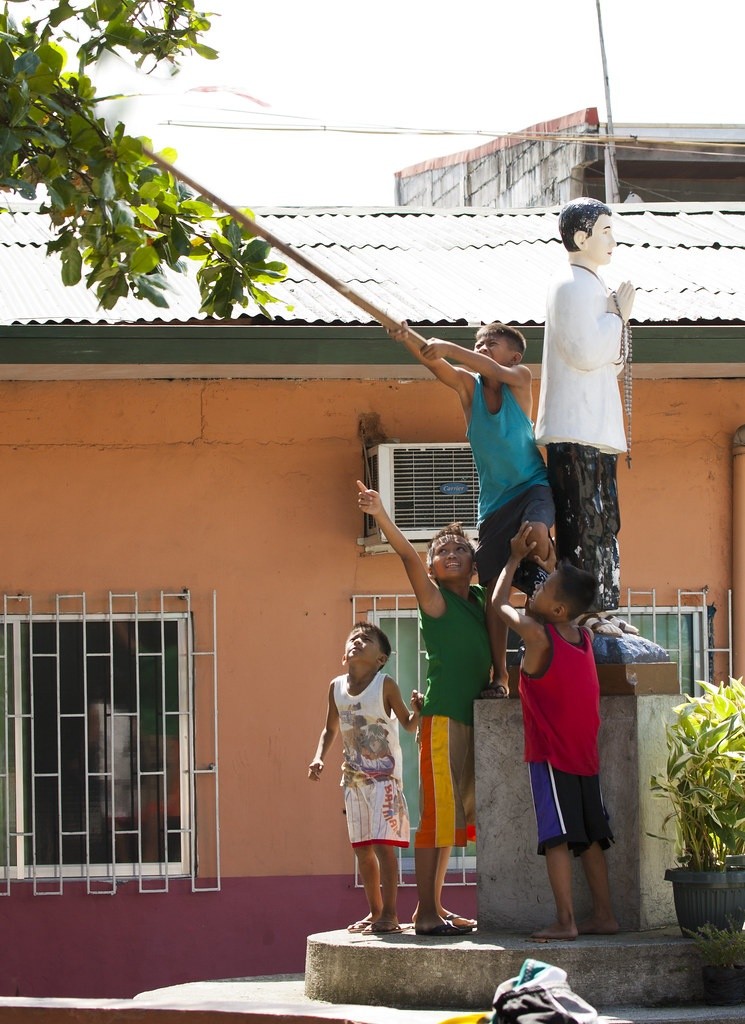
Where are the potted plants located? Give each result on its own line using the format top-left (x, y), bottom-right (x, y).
top-left (681, 923), bottom-right (743, 1007)
top-left (644, 677), bottom-right (742, 940)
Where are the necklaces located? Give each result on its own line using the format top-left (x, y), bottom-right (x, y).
top-left (608, 290), bottom-right (635, 470)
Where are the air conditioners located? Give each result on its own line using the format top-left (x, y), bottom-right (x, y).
top-left (359, 440), bottom-right (483, 551)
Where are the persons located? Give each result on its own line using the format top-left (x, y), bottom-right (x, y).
top-left (379, 323), bottom-right (554, 700)
top-left (354, 477), bottom-right (499, 935)
top-left (537, 197), bottom-right (646, 638)
top-left (492, 524), bottom-right (624, 942)
top-left (308, 622), bottom-right (427, 938)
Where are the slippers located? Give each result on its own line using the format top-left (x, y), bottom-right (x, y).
top-left (578, 613), bottom-right (640, 637)
top-left (348, 920), bottom-right (372, 933)
top-left (443, 913), bottom-right (477, 928)
top-left (415, 920), bottom-right (472, 934)
top-left (361, 920), bottom-right (404, 935)
top-left (480, 685), bottom-right (509, 699)
